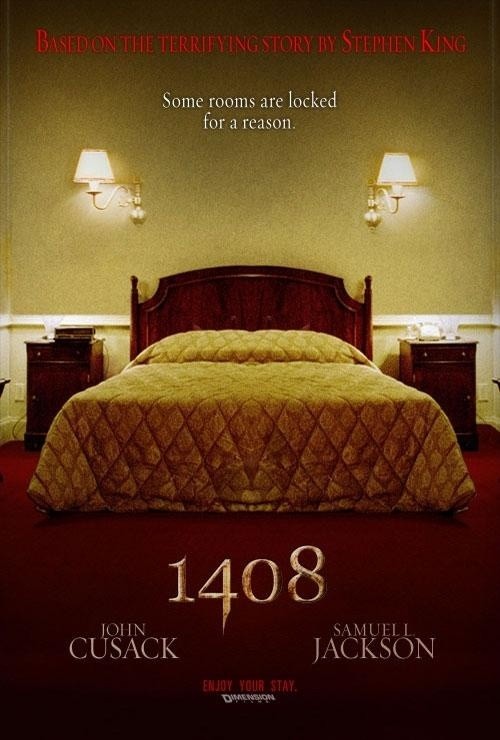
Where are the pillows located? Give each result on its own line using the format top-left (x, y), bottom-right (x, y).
top-left (121, 329), bottom-right (379, 372)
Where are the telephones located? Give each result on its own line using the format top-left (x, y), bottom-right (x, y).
top-left (412, 320), bottom-right (443, 341)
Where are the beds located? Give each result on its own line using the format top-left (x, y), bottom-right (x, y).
top-left (25, 265), bottom-right (477, 521)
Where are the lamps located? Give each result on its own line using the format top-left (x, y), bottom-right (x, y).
top-left (363, 151), bottom-right (418, 231)
top-left (73, 149), bottom-right (147, 228)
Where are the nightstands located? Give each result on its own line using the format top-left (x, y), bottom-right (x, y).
top-left (24, 335), bottom-right (105, 450)
top-left (397, 335), bottom-right (480, 451)
